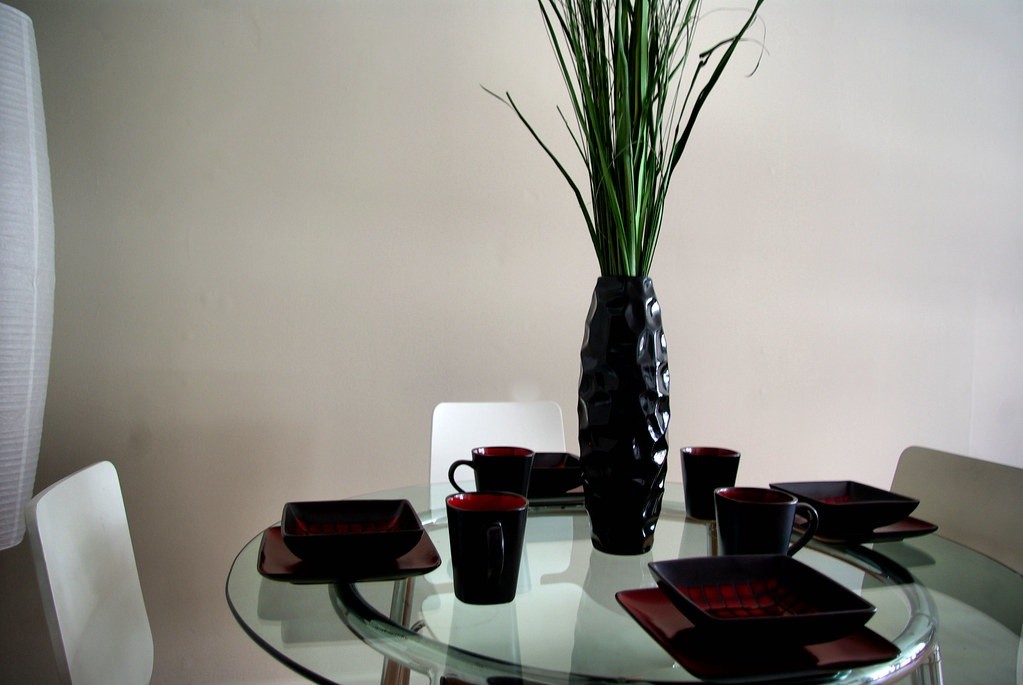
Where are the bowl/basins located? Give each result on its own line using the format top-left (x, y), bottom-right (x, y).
top-left (646, 555), bottom-right (878, 647)
top-left (768, 481), bottom-right (920, 534)
top-left (281, 497), bottom-right (424, 562)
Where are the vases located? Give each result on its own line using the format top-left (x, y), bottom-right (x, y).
top-left (576, 275), bottom-right (670, 556)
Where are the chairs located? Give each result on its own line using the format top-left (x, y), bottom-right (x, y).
top-left (25, 461), bottom-right (154, 684)
top-left (408, 403), bottom-right (673, 685)
top-left (862, 446), bottom-right (1023, 685)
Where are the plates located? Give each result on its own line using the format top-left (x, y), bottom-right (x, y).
top-left (524, 452), bottom-right (585, 496)
top-left (257, 525), bottom-right (441, 583)
top-left (613, 585), bottom-right (902, 684)
top-left (766, 497), bottom-right (939, 549)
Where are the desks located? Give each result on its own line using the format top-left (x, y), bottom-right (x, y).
top-left (226, 478), bottom-right (1022, 683)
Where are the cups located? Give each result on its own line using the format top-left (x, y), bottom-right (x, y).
top-left (711, 486), bottom-right (819, 561)
top-left (446, 490), bottom-right (528, 605)
top-left (447, 446), bottom-right (536, 506)
top-left (680, 445), bottom-right (742, 523)
